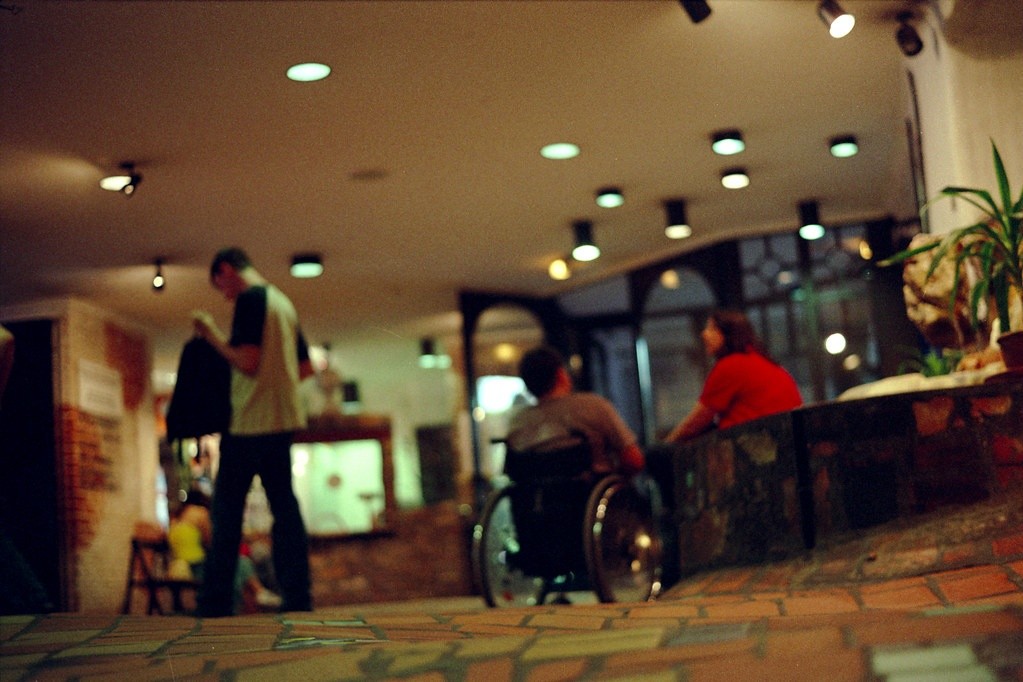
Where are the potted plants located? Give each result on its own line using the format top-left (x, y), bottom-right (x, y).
top-left (877, 230), bottom-right (1023, 369)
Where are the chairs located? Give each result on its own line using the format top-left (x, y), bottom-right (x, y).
top-left (122, 536), bottom-right (200, 617)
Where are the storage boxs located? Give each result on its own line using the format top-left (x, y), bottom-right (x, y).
top-left (679, 0), bottom-right (712, 23)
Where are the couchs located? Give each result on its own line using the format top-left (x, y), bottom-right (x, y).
top-left (670, 401), bottom-right (914, 576)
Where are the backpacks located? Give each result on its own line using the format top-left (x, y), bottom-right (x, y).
top-left (166, 333), bottom-right (232, 443)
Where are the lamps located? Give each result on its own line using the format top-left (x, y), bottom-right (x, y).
top-left (721, 171), bottom-right (749, 189)
top-left (664, 201), bottom-right (692, 239)
top-left (571, 221), bottom-right (601, 262)
top-left (816, 0), bottom-right (855, 39)
top-left (828, 136), bottom-right (859, 158)
top-left (796, 201), bottom-right (825, 241)
top-left (894, 24), bottom-right (923, 57)
top-left (710, 130), bottom-right (746, 156)
top-left (290, 254), bottom-right (323, 278)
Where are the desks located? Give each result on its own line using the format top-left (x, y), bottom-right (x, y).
top-left (790, 379), bottom-right (1023, 550)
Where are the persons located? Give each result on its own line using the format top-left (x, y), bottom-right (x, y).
top-left (665, 308), bottom-right (805, 443)
top-left (191, 245), bottom-right (314, 618)
top-left (504, 346), bottom-right (646, 474)
top-left (169, 501), bottom-right (282, 608)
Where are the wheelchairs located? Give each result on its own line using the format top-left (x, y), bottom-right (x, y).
top-left (469, 428), bottom-right (660, 608)
